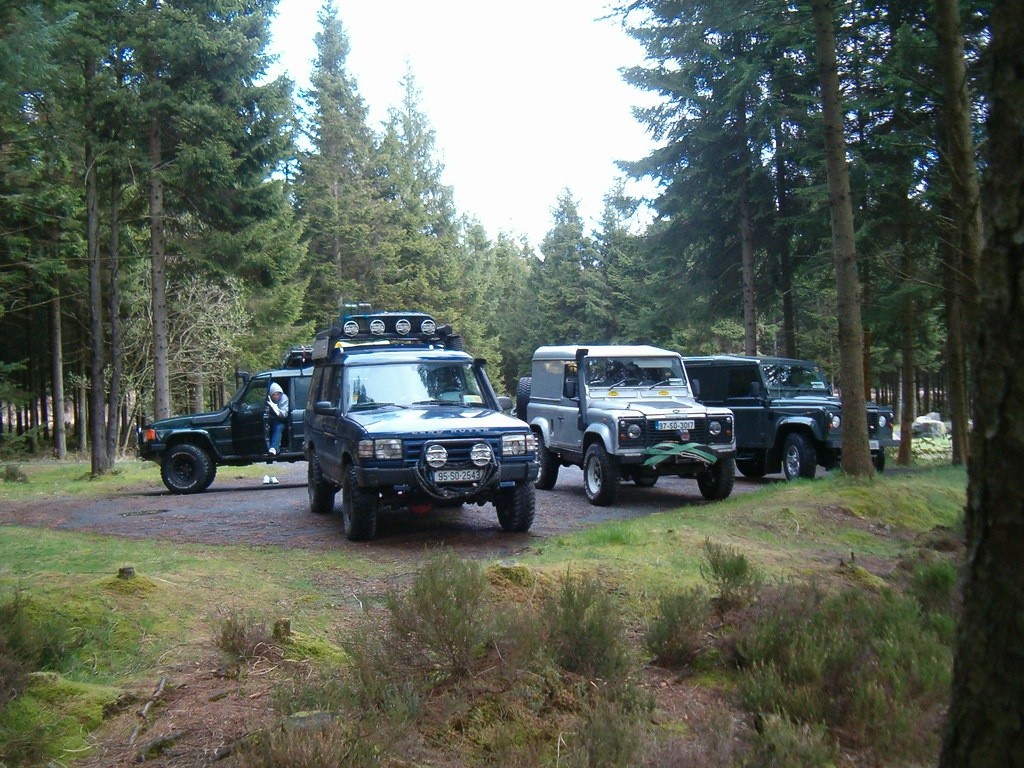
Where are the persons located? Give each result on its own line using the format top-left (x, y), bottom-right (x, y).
top-left (267, 382), bottom-right (289, 455)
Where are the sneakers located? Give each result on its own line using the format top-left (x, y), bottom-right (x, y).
top-left (269, 448), bottom-right (276, 456)
top-left (263, 475), bottom-right (279, 484)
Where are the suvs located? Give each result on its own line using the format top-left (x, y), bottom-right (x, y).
top-left (680, 353), bottom-right (894, 484)
top-left (516, 346), bottom-right (739, 507)
top-left (303, 302), bottom-right (541, 541)
top-left (136, 345), bottom-right (316, 494)
